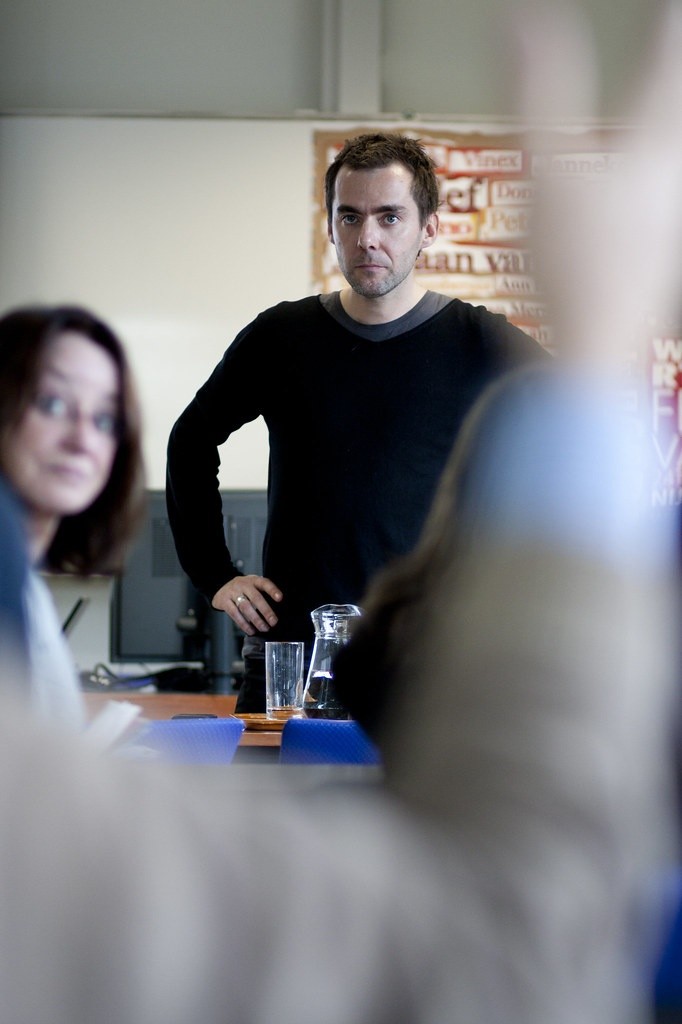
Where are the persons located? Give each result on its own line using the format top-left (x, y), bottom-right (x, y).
top-left (0, 305), bottom-right (149, 570)
top-left (165, 133), bottom-right (555, 713)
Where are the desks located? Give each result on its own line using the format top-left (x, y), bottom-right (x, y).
top-left (85, 694), bottom-right (308, 746)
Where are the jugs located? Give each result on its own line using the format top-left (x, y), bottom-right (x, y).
top-left (304, 604), bottom-right (367, 720)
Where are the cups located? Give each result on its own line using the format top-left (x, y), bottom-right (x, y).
top-left (265, 642), bottom-right (304, 720)
